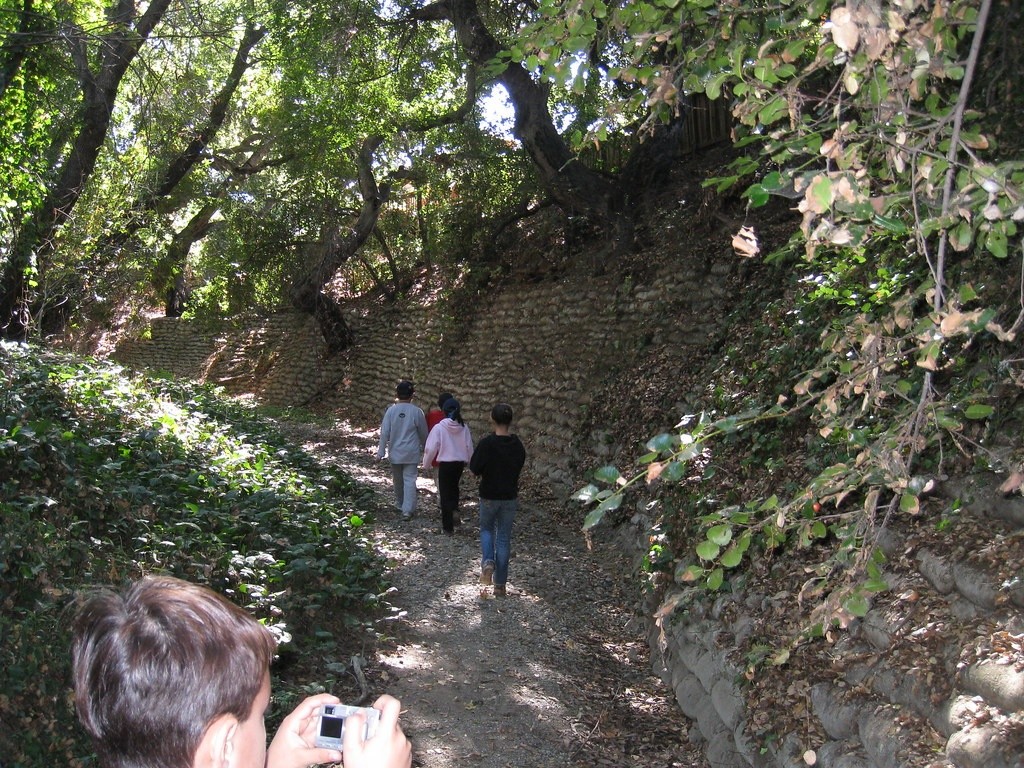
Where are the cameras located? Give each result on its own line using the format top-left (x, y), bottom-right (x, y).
top-left (314, 704), bottom-right (380, 752)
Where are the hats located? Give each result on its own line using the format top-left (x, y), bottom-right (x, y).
top-left (396, 382), bottom-right (415, 399)
top-left (439, 393), bottom-right (453, 405)
top-left (443, 398), bottom-right (460, 413)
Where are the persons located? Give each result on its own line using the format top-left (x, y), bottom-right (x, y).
top-left (469, 403), bottom-right (526, 593)
top-left (74, 573), bottom-right (413, 768)
top-left (373, 381), bottom-right (474, 536)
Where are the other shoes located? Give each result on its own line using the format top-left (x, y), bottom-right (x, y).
top-left (401, 514), bottom-right (410, 521)
top-left (479, 561), bottom-right (494, 586)
top-left (452, 511), bottom-right (461, 526)
top-left (394, 501), bottom-right (402, 510)
top-left (494, 584), bottom-right (506, 595)
top-left (443, 529), bottom-right (454, 536)
